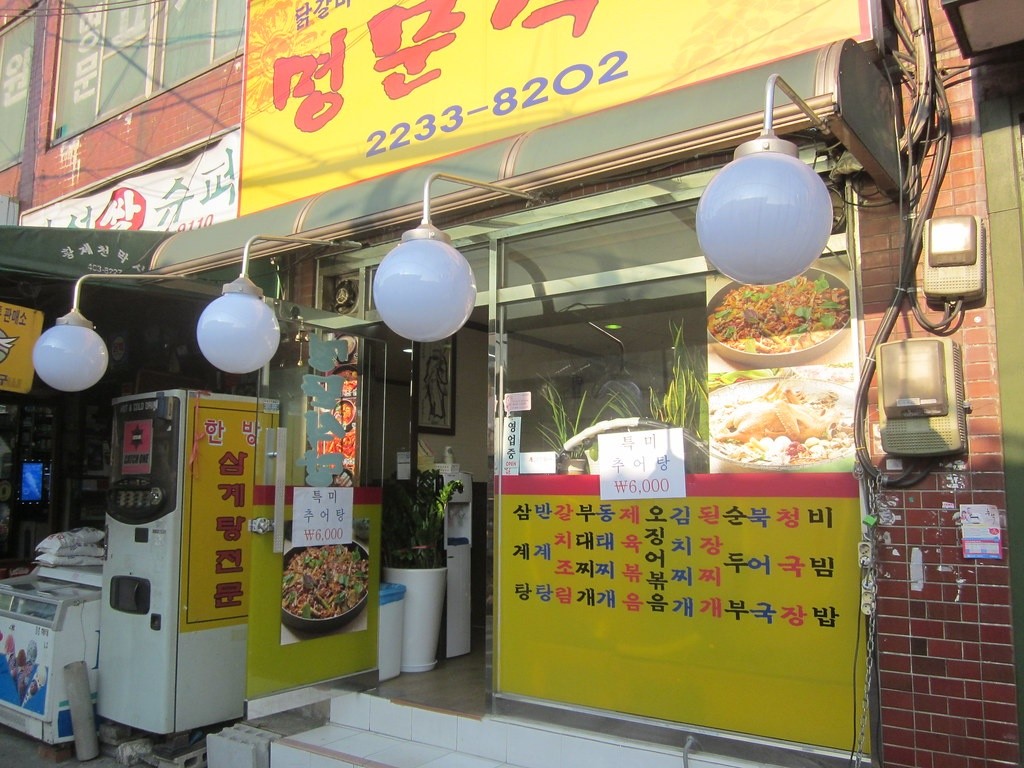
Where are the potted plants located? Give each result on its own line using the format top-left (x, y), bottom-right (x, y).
top-left (387, 466), bottom-right (465, 673)
top-left (542, 383), bottom-right (620, 475)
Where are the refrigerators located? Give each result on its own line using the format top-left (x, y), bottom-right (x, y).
top-left (0, 572), bottom-right (103, 743)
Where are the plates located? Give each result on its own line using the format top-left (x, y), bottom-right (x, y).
top-left (282, 539), bottom-right (369, 629)
top-left (708, 377), bottom-right (858, 470)
top-left (707, 267), bottom-right (850, 366)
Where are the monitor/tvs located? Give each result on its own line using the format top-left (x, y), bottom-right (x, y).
top-left (20, 462), bottom-right (43, 501)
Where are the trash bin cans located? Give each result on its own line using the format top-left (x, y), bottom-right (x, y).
top-left (379, 581), bottom-right (408, 683)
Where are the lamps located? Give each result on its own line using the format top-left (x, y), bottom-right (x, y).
top-left (695, 74), bottom-right (832, 288)
top-left (32, 274), bottom-right (199, 392)
top-left (197, 237), bottom-right (362, 374)
top-left (372, 172), bottom-right (540, 342)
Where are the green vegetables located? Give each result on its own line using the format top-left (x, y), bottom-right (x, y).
top-left (714, 273), bottom-right (838, 352)
top-left (282, 545), bottom-right (364, 619)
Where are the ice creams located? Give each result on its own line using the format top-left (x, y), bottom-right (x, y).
top-left (5, 635), bottom-right (47, 709)
